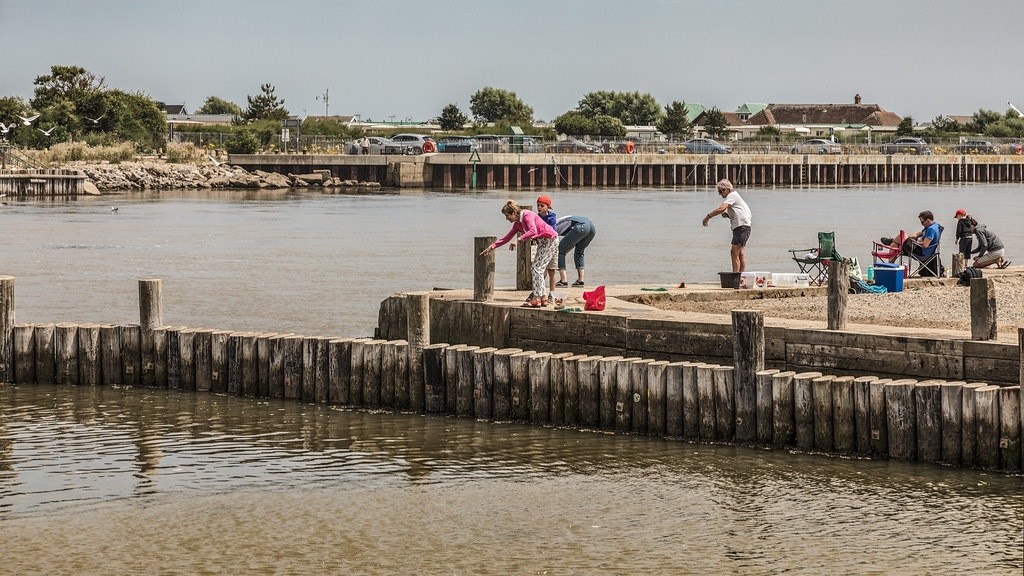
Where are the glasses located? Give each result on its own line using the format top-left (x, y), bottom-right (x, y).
top-left (921, 220), bottom-right (926, 225)
top-left (719, 189), bottom-right (726, 193)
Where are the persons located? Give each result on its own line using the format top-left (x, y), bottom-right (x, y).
top-left (954, 209), bottom-right (972, 266)
top-left (361, 135), bottom-right (371, 155)
top-left (971, 218), bottom-right (1012, 270)
top-left (478, 200), bottom-right (559, 307)
top-left (703, 178), bottom-right (752, 272)
top-left (525, 196), bottom-right (558, 301)
top-left (882, 210), bottom-right (940, 263)
top-left (555, 214), bottom-right (596, 287)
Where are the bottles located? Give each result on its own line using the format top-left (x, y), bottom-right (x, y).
top-left (868, 265), bottom-right (873, 280)
top-left (903, 261), bottom-right (908, 279)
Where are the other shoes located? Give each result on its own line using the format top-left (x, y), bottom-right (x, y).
top-left (526, 293), bottom-right (534, 301)
top-left (881, 237), bottom-right (892, 246)
top-left (548, 293), bottom-right (554, 304)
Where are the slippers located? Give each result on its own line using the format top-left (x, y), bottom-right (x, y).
top-left (996, 261), bottom-right (1007, 269)
top-left (1004, 262), bottom-right (1011, 269)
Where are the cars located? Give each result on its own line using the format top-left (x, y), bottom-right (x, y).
top-left (955, 140), bottom-right (994, 154)
top-left (788, 138), bottom-right (842, 155)
top-left (344, 136), bottom-right (413, 156)
top-left (676, 138), bottom-right (733, 154)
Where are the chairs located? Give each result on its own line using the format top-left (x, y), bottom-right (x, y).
top-left (871, 229), bottom-right (907, 265)
top-left (907, 224), bottom-right (945, 279)
top-left (787, 231), bottom-right (843, 287)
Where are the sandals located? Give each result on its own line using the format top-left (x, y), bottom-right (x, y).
top-left (541, 295), bottom-right (548, 306)
top-left (521, 299), bottom-right (541, 308)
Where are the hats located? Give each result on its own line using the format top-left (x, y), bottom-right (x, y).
top-left (954, 210), bottom-right (966, 218)
top-left (537, 196), bottom-right (553, 210)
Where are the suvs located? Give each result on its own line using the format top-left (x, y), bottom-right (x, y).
top-left (388, 133), bottom-right (438, 153)
top-left (879, 137), bottom-right (929, 155)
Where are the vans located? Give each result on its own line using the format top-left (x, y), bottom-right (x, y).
top-left (435, 135), bottom-right (644, 153)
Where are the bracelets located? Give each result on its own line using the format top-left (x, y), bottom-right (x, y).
top-left (708, 214), bottom-right (711, 218)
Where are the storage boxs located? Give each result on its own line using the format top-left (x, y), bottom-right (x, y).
top-left (771, 272), bottom-right (810, 288)
top-left (740, 271), bottom-right (771, 289)
top-left (873, 265), bottom-right (905, 293)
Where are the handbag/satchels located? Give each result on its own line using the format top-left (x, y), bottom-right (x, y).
top-left (583, 286), bottom-right (606, 311)
top-left (961, 267), bottom-right (982, 286)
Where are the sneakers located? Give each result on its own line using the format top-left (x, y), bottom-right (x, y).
top-left (571, 280), bottom-right (584, 286)
top-left (555, 280), bottom-right (568, 288)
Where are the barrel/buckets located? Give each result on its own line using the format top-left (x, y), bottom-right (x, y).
top-left (718, 272), bottom-right (741, 288)
